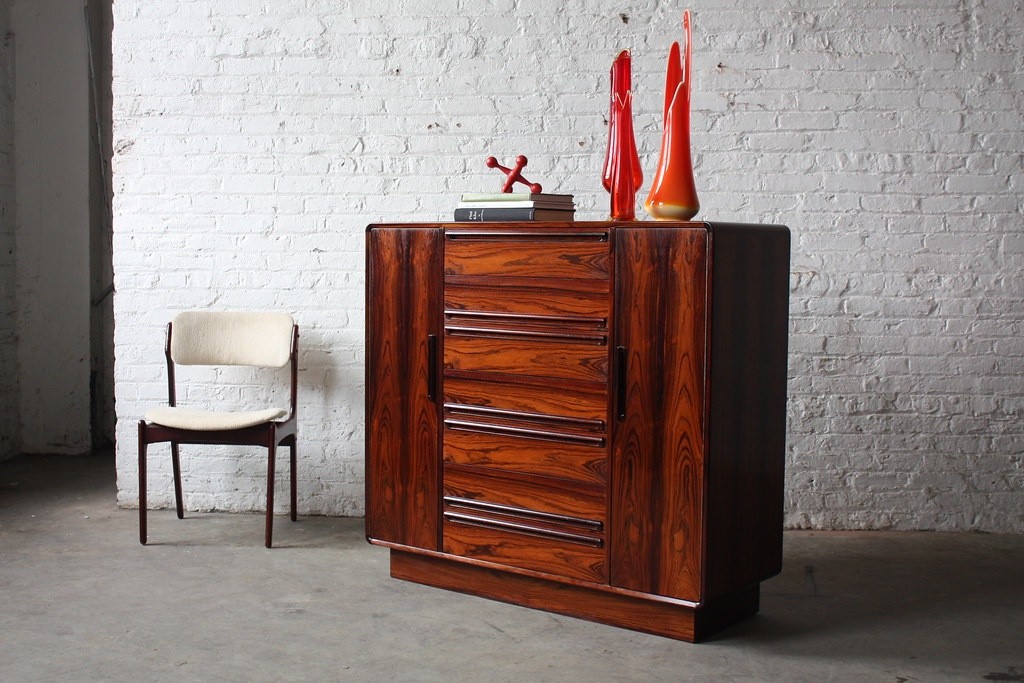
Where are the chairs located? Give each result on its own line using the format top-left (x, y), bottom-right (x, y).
top-left (135, 310), bottom-right (300, 548)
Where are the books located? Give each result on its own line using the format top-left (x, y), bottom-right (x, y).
top-left (454, 193), bottom-right (576, 221)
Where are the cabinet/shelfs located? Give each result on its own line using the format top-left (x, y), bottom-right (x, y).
top-left (361, 218), bottom-right (793, 650)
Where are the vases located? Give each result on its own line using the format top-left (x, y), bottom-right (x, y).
top-left (599, 46), bottom-right (644, 219)
top-left (644, 7), bottom-right (701, 221)
